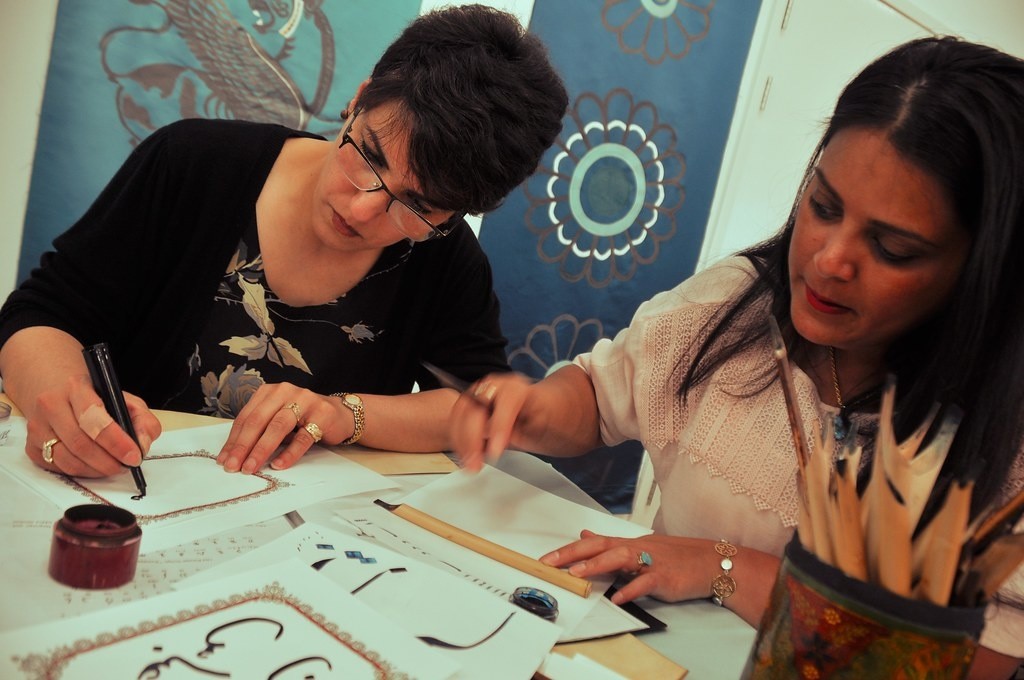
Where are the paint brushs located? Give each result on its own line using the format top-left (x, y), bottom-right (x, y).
top-left (759, 303), bottom-right (999, 625)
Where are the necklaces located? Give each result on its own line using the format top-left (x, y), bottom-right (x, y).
top-left (828, 346), bottom-right (850, 441)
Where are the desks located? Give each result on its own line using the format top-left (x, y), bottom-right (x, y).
top-left (0, 386), bottom-right (759, 680)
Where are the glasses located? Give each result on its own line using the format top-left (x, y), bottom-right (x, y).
top-left (336, 101), bottom-right (467, 242)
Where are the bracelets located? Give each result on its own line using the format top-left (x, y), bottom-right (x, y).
top-left (712, 540), bottom-right (737, 607)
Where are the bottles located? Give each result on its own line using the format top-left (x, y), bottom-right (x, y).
top-left (510, 587), bottom-right (559, 622)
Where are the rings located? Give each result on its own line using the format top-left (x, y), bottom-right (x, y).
top-left (301, 421), bottom-right (323, 443)
top-left (635, 550), bottom-right (653, 574)
top-left (475, 382), bottom-right (497, 401)
top-left (41, 438), bottom-right (61, 464)
top-left (281, 401), bottom-right (302, 423)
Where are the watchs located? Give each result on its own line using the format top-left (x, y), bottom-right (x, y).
top-left (329, 392), bottom-right (365, 447)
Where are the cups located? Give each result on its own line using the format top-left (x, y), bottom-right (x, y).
top-left (48, 504), bottom-right (142, 589)
top-left (739, 545), bottom-right (990, 680)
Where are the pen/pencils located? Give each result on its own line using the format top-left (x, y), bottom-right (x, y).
top-left (418, 355), bottom-right (501, 415)
top-left (370, 490), bottom-right (595, 601)
top-left (80, 336), bottom-right (150, 499)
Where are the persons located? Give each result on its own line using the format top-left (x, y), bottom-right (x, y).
top-left (449, 35), bottom-right (1024, 680)
top-left (0, 3), bottom-right (569, 479)
